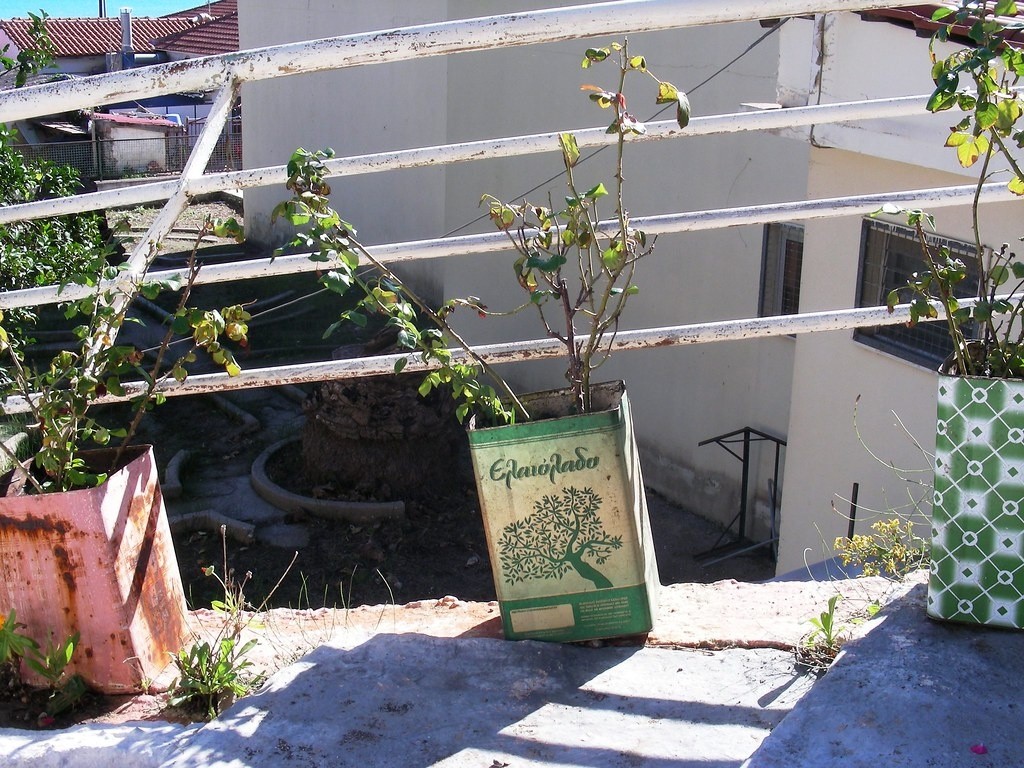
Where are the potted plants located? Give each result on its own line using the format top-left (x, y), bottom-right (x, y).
top-left (887, 0), bottom-right (1024, 629)
top-left (270, 36), bottom-right (689, 645)
top-left (0, 12), bottom-right (257, 694)
